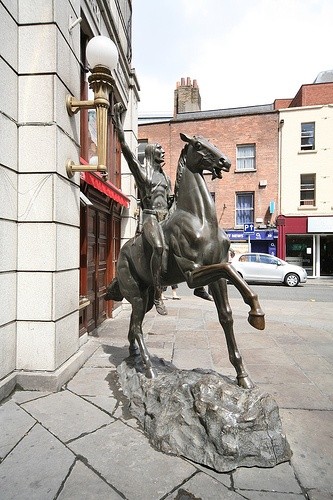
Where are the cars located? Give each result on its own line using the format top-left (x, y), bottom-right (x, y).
top-left (229, 252), bottom-right (308, 288)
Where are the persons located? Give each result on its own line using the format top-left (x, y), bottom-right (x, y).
top-left (112, 102), bottom-right (216, 318)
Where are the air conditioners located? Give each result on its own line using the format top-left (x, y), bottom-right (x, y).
top-left (259, 180), bottom-right (268, 187)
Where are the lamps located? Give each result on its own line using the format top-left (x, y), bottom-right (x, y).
top-left (65, 36), bottom-right (121, 182)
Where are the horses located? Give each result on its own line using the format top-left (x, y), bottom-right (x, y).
top-left (116, 132), bottom-right (267, 389)
